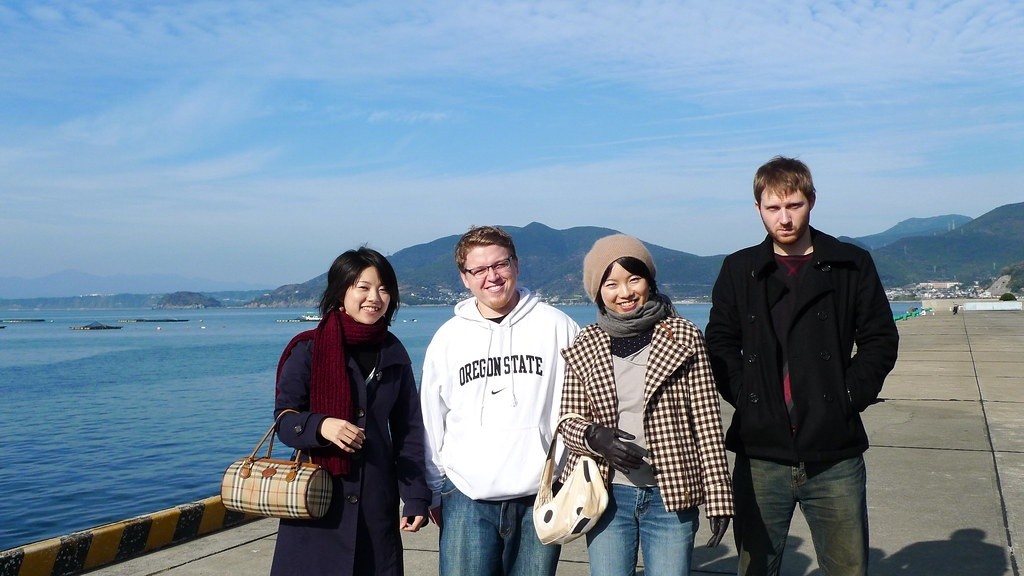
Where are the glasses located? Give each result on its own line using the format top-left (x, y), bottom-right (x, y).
top-left (464, 254), bottom-right (513, 278)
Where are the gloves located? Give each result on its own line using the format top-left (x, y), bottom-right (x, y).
top-left (584, 421), bottom-right (643, 474)
top-left (706, 516), bottom-right (730, 548)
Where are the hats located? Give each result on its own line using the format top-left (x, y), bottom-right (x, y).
top-left (582, 234), bottom-right (656, 304)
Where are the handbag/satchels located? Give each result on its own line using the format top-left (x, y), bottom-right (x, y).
top-left (532, 413), bottom-right (608, 545)
top-left (221, 408), bottom-right (333, 519)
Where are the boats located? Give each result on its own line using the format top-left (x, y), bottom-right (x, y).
top-left (303, 314), bottom-right (323, 322)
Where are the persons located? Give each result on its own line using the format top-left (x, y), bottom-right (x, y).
top-left (269, 247), bottom-right (432, 576)
top-left (420, 226), bottom-right (581, 576)
top-left (558, 234), bottom-right (738, 576)
top-left (705, 155), bottom-right (900, 576)
